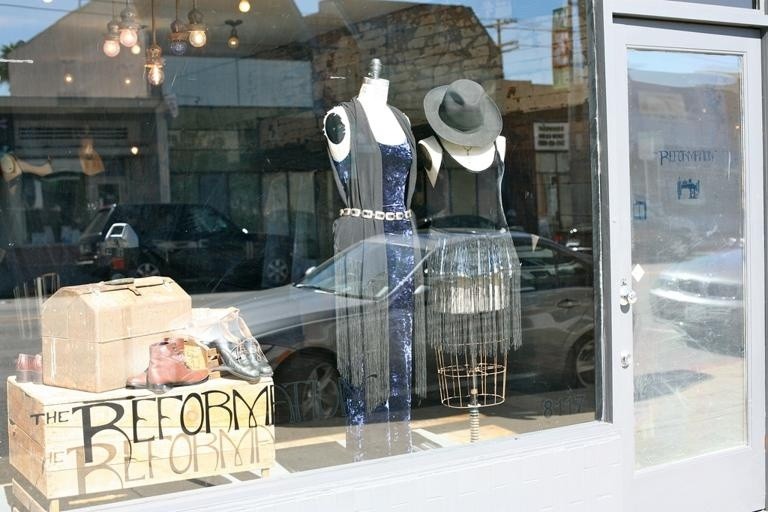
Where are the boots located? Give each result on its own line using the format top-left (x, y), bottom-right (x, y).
top-left (125, 337), bottom-right (210, 392)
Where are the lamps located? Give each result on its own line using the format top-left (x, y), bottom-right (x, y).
top-left (103, 0), bottom-right (251, 88)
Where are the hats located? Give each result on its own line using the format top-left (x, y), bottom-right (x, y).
top-left (423, 78), bottom-right (504, 148)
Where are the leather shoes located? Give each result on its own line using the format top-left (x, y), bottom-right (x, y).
top-left (209, 319), bottom-right (260, 382)
top-left (227, 316), bottom-right (274, 377)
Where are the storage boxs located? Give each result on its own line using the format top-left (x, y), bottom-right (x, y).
top-left (7, 375), bottom-right (274, 499)
top-left (42, 276), bottom-right (192, 393)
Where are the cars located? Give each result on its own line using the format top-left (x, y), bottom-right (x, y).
top-left (550, 225), bottom-right (593, 256)
top-left (187, 226), bottom-right (594, 423)
top-left (650, 249), bottom-right (744, 356)
top-left (415, 213), bottom-right (496, 230)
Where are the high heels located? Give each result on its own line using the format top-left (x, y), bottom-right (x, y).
top-left (15, 351), bottom-right (42, 386)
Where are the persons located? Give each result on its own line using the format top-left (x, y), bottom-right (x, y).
top-left (419, 79), bottom-right (519, 355)
top-left (324, 59), bottom-right (426, 461)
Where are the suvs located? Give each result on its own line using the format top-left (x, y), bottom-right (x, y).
top-left (74, 203), bottom-right (296, 290)
